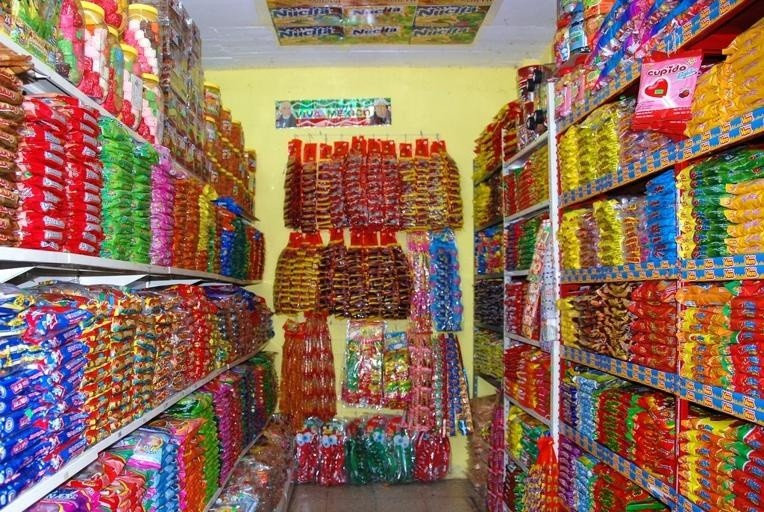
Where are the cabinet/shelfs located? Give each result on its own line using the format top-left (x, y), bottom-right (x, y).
top-left (0, 30), bottom-right (299, 512)
top-left (473, 0), bottom-right (763, 511)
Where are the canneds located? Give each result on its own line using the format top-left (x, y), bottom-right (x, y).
top-left (129, 3), bottom-right (163, 77)
top-left (104, 22), bottom-right (124, 113)
top-left (202, 83), bottom-right (255, 216)
top-left (79, 0), bottom-right (110, 104)
top-left (139, 71), bottom-right (165, 149)
top-left (121, 41), bottom-right (143, 131)
